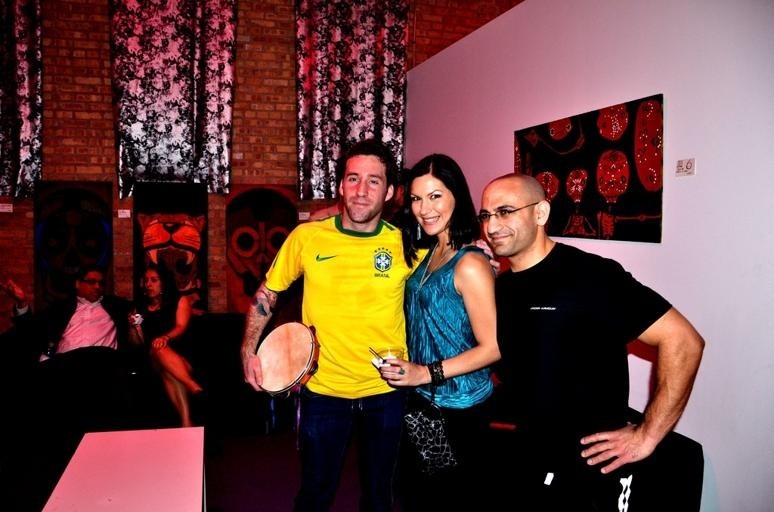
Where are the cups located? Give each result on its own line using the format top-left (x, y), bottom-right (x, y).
top-left (378, 346), bottom-right (404, 366)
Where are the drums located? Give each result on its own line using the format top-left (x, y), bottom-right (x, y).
top-left (256, 321), bottom-right (320, 397)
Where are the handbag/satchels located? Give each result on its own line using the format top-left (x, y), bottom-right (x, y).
top-left (404, 406), bottom-right (459, 475)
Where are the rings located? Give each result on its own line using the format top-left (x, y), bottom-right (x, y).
top-left (399, 368), bottom-right (405, 374)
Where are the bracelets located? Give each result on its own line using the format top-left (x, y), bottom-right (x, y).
top-left (426, 360), bottom-right (445, 387)
top-left (162, 334), bottom-right (171, 340)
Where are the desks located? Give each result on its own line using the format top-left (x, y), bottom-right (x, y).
top-left (41, 426), bottom-right (207, 512)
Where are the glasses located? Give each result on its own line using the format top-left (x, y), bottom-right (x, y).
top-left (475, 202), bottom-right (539, 223)
top-left (81, 279), bottom-right (107, 288)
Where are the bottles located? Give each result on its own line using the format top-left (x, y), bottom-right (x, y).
top-left (39, 341), bottom-right (55, 362)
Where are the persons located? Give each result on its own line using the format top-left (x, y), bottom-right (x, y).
top-left (125, 255), bottom-right (206, 431)
top-left (0, 262), bottom-right (143, 464)
top-left (237, 138), bottom-right (502, 510)
top-left (381, 168), bottom-right (412, 229)
top-left (479, 171), bottom-right (706, 511)
top-left (372, 150), bottom-right (504, 511)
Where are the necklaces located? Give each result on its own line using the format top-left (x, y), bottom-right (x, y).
top-left (414, 240), bottom-right (455, 297)
